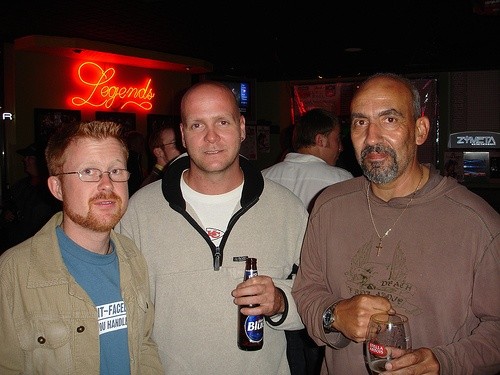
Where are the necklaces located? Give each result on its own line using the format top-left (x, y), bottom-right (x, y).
top-left (366, 170), bottom-right (424, 256)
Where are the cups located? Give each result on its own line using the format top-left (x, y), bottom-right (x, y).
top-left (363, 312), bottom-right (412, 375)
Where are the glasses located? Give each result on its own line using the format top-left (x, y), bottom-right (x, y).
top-left (154, 139), bottom-right (180, 148)
top-left (55, 168), bottom-right (131, 183)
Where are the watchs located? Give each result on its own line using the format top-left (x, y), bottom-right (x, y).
top-left (323, 304), bottom-right (340, 333)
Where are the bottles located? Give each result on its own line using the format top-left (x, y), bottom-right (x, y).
top-left (237, 258), bottom-right (264, 352)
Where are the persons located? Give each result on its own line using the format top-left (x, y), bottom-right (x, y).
top-left (110, 81), bottom-right (311, 375)
top-left (0, 121), bottom-right (166, 375)
top-left (290, 72), bottom-right (500, 375)
top-left (139, 123), bottom-right (188, 190)
top-left (0, 141), bottom-right (63, 254)
top-left (259, 108), bottom-right (355, 212)
